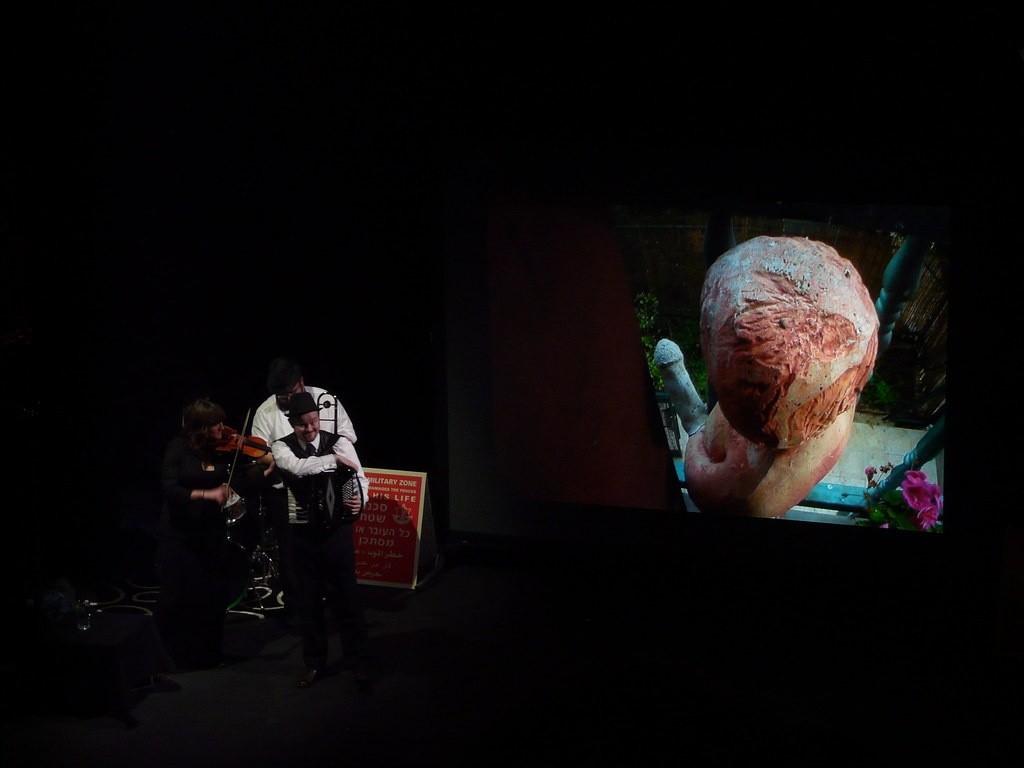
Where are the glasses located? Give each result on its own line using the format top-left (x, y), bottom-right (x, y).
top-left (275, 385), bottom-right (306, 401)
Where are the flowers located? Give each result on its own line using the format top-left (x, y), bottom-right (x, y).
top-left (848, 463), bottom-right (943, 532)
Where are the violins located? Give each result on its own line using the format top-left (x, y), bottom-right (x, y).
top-left (209, 405), bottom-right (277, 512)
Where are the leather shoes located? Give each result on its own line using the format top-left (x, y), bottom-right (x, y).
top-left (297, 664), bottom-right (327, 689)
top-left (354, 660), bottom-right (376, 683)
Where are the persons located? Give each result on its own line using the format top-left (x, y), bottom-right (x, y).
top-left (155, 399), bottom-right (275, 674)
top-left (250, 359), bottom-right (359, 638)
top-left (270, 393), bottom-right (392, 690)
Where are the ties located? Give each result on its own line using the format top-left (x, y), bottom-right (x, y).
top-left (305, 442), bottom-right (318, 466)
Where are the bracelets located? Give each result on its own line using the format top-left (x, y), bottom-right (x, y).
top-left (200, 488), bottom-right (205, 500)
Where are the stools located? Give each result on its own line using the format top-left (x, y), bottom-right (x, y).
top-left (41, 612), bottom-right (181, 725)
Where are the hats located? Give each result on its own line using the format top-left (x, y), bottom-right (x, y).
top-left (286, 391), bottom-right (326, 420)
